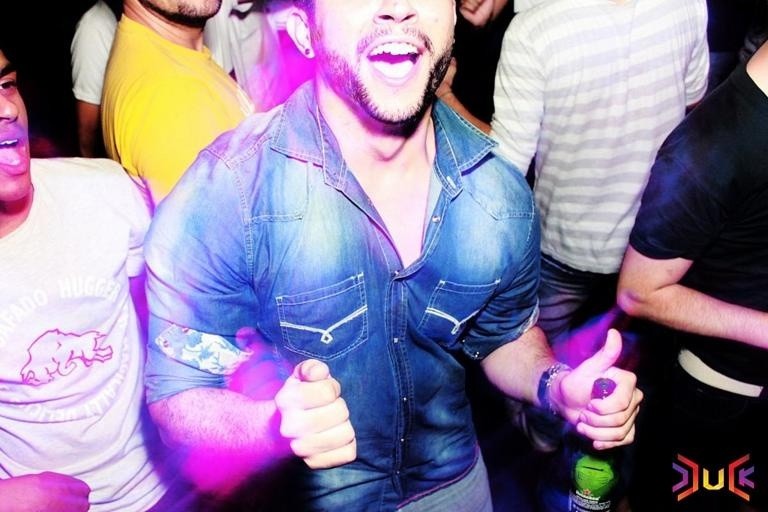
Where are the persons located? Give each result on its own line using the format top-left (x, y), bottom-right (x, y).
top-left (1, 0), bottom-right (768, 511)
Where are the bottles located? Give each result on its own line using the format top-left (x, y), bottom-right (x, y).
top-left (568, 378), bottom-right (623, 512)
